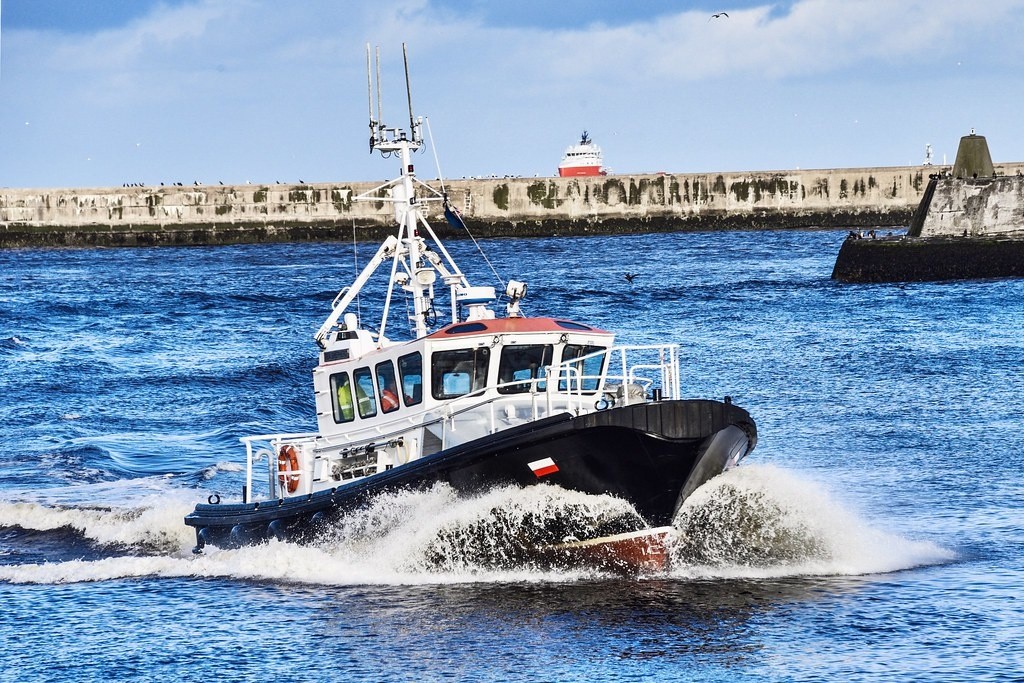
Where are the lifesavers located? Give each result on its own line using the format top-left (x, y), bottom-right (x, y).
top-left (276, 446), bottom-right (300, 493)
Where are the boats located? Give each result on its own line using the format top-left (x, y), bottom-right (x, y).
top-left (557, 129), bottom-right (604, 178)
top-left (184, 41), bottom-right (760, 587)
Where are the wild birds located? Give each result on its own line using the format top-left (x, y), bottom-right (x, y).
top-left (625, 274), bottom-right (638, 283)
top-left (708, 12), bottom-right (729, 23)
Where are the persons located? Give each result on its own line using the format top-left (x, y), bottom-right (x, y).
top-left (337, 374), bottom-right (372, 420)
top-left (381, 374), bottom-right (413, 413)
